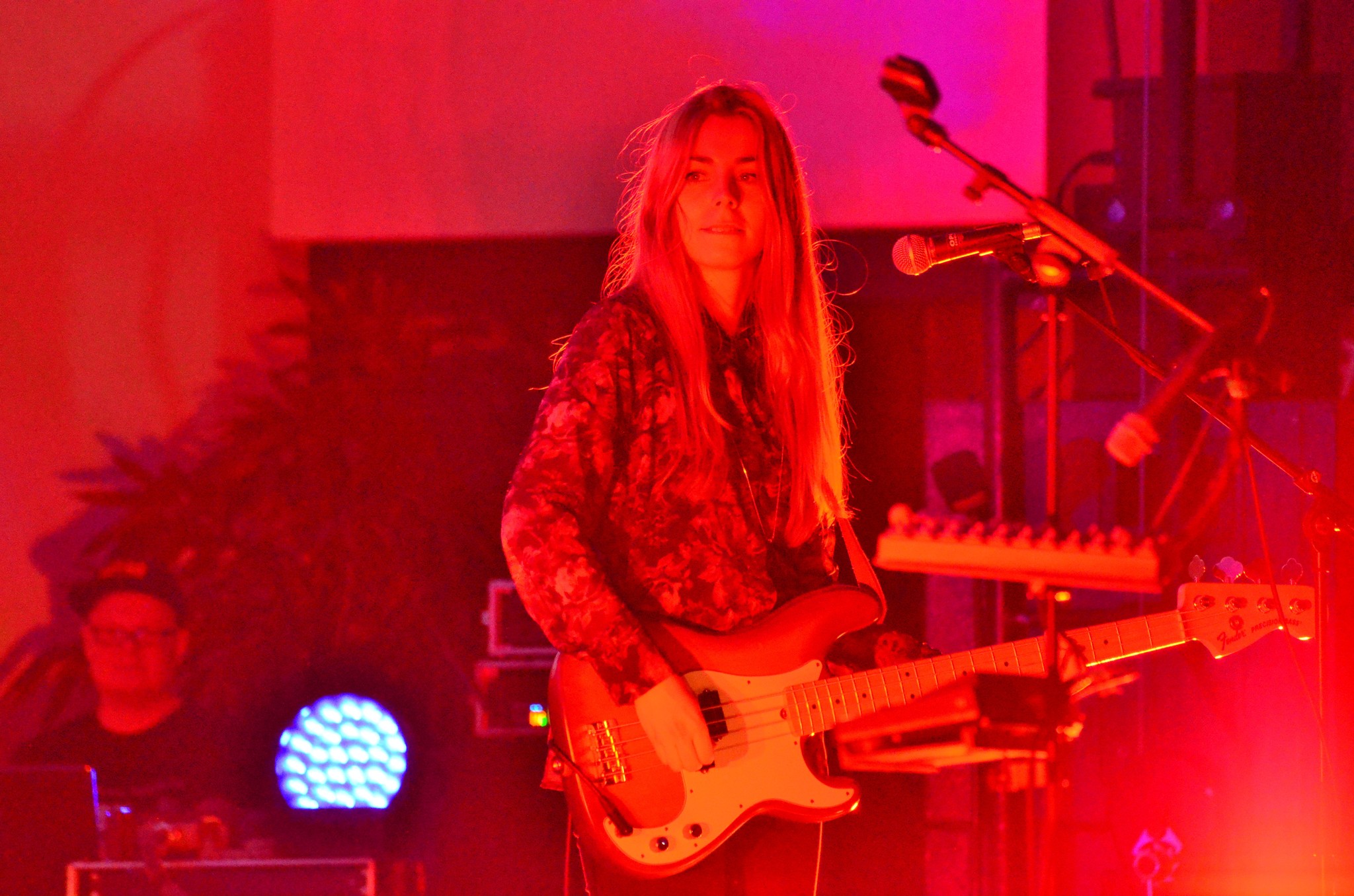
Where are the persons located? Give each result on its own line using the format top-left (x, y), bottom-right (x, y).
top-left (12, 554), bottom-right (241, 830)
top-left (497, 75), bottom-right (950, 894)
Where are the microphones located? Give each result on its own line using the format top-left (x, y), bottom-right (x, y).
top-left (1106, 296), bottom-right (1258, 468)
top-left (892, 218), bottom-right (1056, 276)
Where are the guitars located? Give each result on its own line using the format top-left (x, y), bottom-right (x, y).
top-left (547, 555), bottom-right (1313, 881)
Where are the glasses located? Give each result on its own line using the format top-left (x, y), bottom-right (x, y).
top-left (81, 615), bottom-right (179, 646)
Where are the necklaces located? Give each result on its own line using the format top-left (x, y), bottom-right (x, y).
top-left (724, 423), bottom-right (788, 544)
